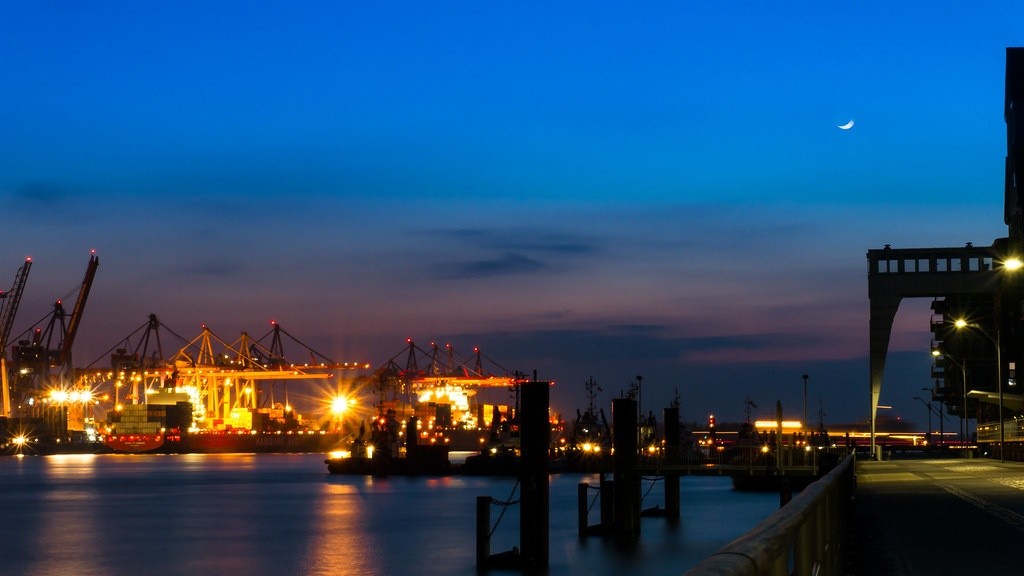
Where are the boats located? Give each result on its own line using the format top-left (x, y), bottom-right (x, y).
top-left (323, 408), bottom-right (452, 479)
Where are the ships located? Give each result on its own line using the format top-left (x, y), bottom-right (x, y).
top-left (102, 312), bottom-right (565, 453)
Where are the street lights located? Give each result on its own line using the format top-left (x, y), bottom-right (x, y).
top-left (912, 320), bottom-right (1005, 463)
top-left (636, 376), bottom-right (643, 454)
top-left (803, 374), bottom-right (808, 439)
